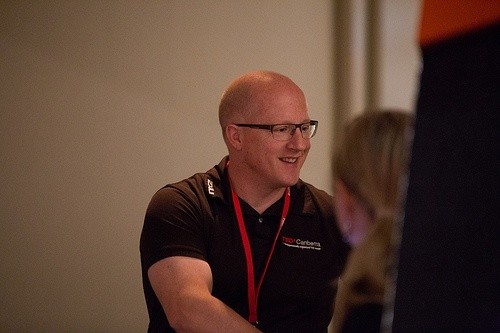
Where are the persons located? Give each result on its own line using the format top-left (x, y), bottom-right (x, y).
top-left (380, 0), bottom-right (500, 333)
top-left (328, 109), bottom-right (417, 333)
top-left (138, 70), bottom-right (355, 333)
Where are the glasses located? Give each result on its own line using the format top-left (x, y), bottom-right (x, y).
top-left (234, 119), bottom-right (319, 141)
